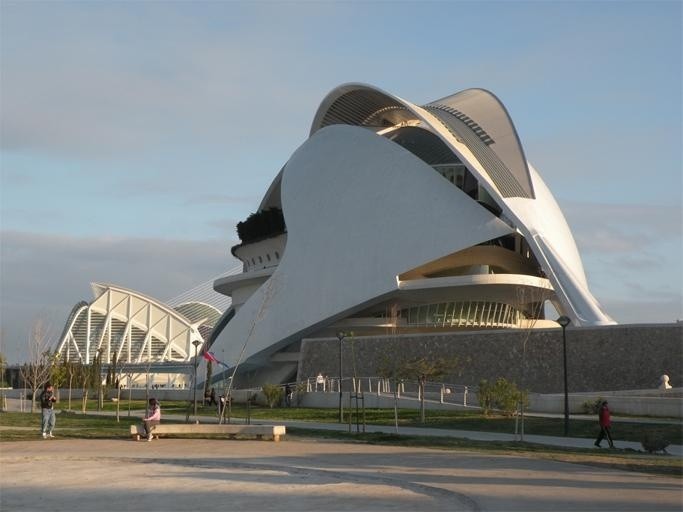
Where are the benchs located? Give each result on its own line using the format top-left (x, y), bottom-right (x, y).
top-left (130, 424), bottom-right (286, 441)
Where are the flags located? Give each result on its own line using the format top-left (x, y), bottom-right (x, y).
top-left (202, 351), bottom-right (230, 370)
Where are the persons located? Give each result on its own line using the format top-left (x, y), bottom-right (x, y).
top-left (39, 381), bottom-right (57, 439)
top-left (593, 400), bottom-right (615, 450)
top-left (139, 396), bottom-right (159, 442)
top-left (282, 381), bottom-right (293, 408)
top-left (204, 386), bottom-right (233, 417)
top-left (315, 369), bottom-right (324, 390)
top-left (324, 372), bottom-right (330, 392)
top-left (119, 383), bottom-right (186, 390)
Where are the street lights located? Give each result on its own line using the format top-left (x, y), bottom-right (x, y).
top-left (191, 339), bottom-right (203, 417)
top-left (335, 329), bottom-right (348, 422)
top-left (555, 314), bottom-right (572, 434)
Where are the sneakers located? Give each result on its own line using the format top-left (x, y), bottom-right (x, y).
top-left (42, 432), bottom-right (55, 440)
top-left (146, 432), bottom-right (154, 442)
